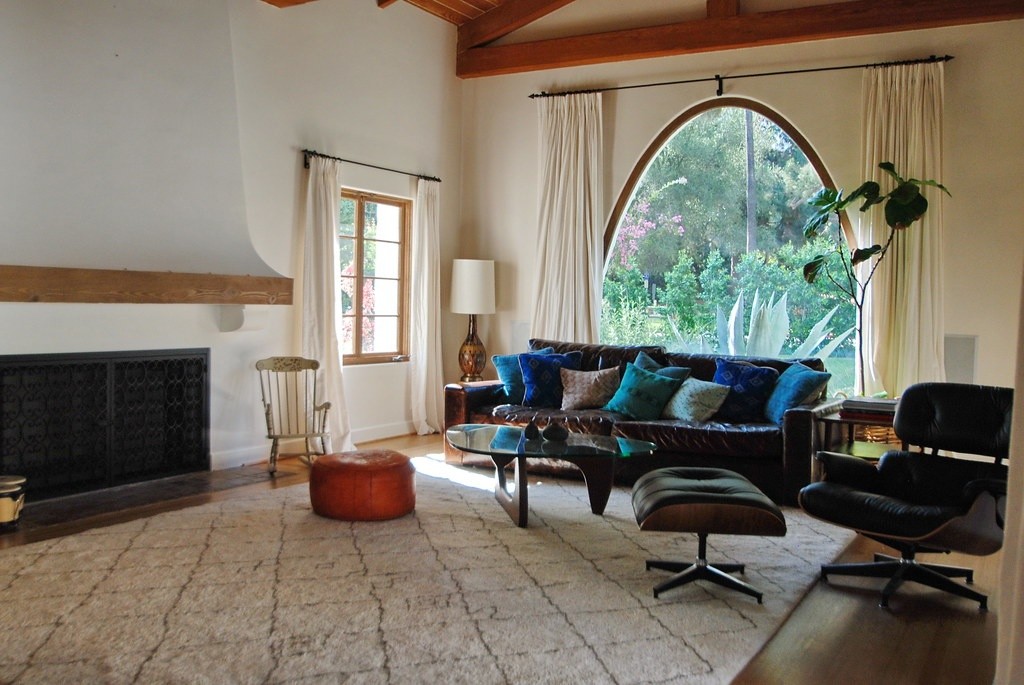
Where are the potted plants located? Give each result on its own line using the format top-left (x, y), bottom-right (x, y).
top-left (803, 162), bottom-right (953, 465)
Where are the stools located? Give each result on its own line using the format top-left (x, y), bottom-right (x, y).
top-left (631, 467), bottom-right (786, 605)
top-left (310, 451), bottom-right (415, 521)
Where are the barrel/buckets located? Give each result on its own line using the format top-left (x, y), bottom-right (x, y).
top-left (0, 475), bottom-right (27, 531)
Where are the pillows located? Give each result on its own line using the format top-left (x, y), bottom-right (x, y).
top-left (601, 362), bottom-right (682, 420)
top-left (490, 347), bottom-right (555, 405)
top-left (764, 362), bottom-right (832, 429)
top-left (560, 365), bottom-right (621, 410)
top-left (660, 376), bottom-right (731, 421)
top-left (712, 357), bottom-right (779, 423)
top-left (633, 351), bottom-right (692, 380)
top-left (518, 351), bottom-right (583, 407)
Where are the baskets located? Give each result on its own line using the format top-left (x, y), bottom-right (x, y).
top-left (841, 419), bottom-right (902, 469)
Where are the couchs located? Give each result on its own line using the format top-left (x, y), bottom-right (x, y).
top-left (444, 338), bottom-right (827, 510)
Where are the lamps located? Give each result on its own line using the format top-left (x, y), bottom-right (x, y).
top-left (450, 259), bottom-right (495, 382)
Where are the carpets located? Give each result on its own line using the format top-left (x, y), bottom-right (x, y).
top-left (0, 453), bottom-right (857, 685)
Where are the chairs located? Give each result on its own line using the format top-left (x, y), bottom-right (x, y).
top-left (255, 356), bottom-right (331, 478)
top-left (797, 382), bottom-right (1014, 610)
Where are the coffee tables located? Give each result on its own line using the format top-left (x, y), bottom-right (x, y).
top-left (447, 423), bottom-right (657, 529)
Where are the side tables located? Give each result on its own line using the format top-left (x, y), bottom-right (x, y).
top-left (819, 412), bottom-right (926, 471)
